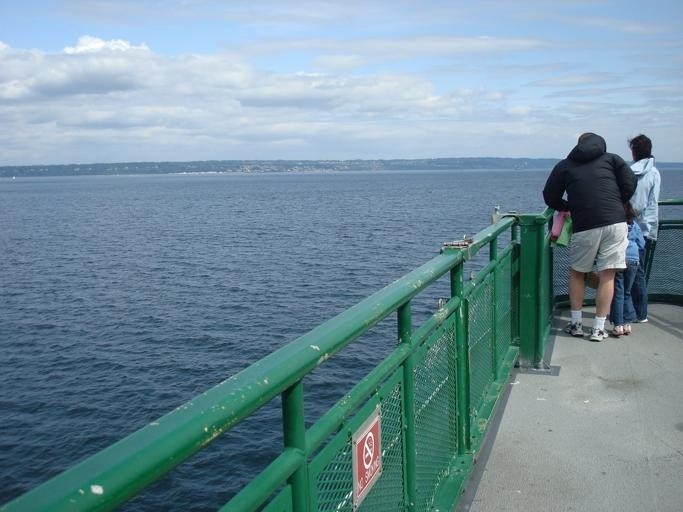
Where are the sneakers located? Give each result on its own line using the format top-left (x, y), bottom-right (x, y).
top-left (565, 321), bottom-right (632, 341)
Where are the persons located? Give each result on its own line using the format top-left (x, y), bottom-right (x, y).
top-left (543, 133), bottom-right (637, 342)
top-left (629, 135), bottom-right (661, 323)
top-left (611, 201), bottom-right (645, 336)
